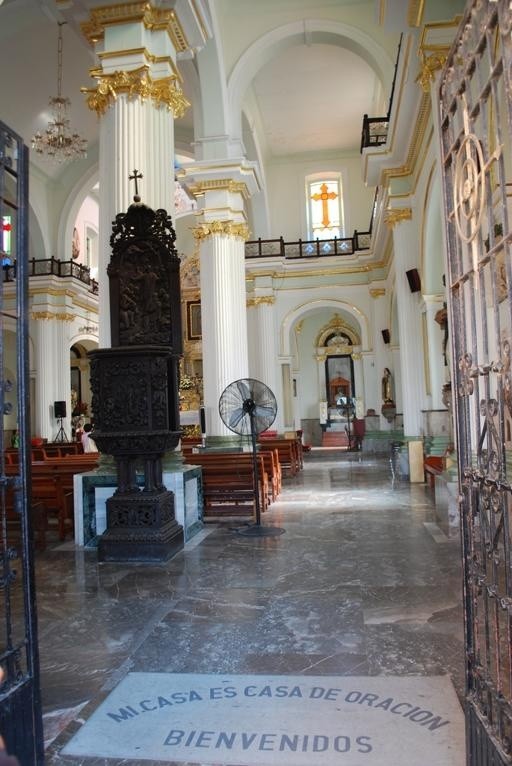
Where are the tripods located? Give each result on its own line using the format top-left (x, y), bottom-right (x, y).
top-left (55, 418), bottom-right (69, 443)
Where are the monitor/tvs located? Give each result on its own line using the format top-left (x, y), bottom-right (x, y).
top-left (336, 396), bottom-right (347, 406)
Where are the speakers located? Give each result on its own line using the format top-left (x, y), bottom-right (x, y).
top-left (405, 268), bottom-right (421, 293)
top-left (381, 329), bottom-right (390, 344)
top-left (54, 401), bottom-right (67, 418)
top-left (199, 406), bottom-right (206, 438)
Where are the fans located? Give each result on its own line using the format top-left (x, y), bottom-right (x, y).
top-left (336, 396), bottom-right (359, 453)
top-left (218, 379), bottom-right (285, 536)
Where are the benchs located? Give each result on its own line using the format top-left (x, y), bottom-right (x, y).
top-left (4, 453), bottom-right (99, 542)
top-left (183, 438), bottom-right (304, 519)
top-left (424, 446), bottom-right (453, 489)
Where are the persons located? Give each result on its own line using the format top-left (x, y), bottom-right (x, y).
top-left (81, 424), bottom-right (98, 454)
top-left (10, 430), bottom-right (20, 449)
top-left (383, 368), bottom-right (393, 406)
top-left (2, 252), bottom-right (17, 282)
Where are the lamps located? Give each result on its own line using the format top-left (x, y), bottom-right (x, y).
top-left (52, 400), bottom-right (69, 444)
top-left (29, 18), bottom-right (89, 165)
